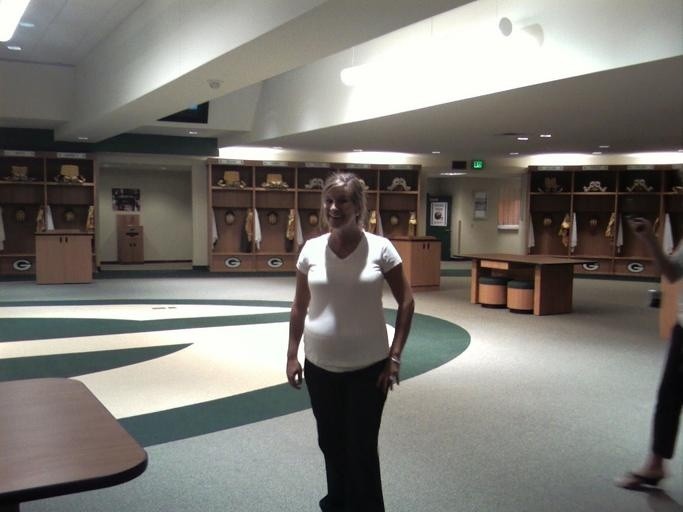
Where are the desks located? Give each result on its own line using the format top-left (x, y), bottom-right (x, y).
top-left (35, 233), bottom-right (94, 284)
top-left (390, 236), bottom-right (442, 288)
top-left (454, 252), bottom-right (597, 317)
top-left (0, 376), bottom-right (148, 512)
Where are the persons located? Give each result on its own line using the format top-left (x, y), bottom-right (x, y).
top-left (286, 171), bottom-right (415, 512)
top-left (614, 216), bottom-right (683, 490)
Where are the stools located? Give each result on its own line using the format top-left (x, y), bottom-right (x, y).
top-left (477, 277), bottom-right (511, 308)
top-left (505, 281), bottom-right (535, 312)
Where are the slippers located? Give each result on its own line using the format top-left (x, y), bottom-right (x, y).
top-left (614, 468), bottom-right (664, 488)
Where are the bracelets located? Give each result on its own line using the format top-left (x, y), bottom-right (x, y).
top-left (389, 356), bottom-right (401, 366)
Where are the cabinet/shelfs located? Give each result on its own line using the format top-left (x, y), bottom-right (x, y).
top-left (0, 151), bottom-right (97, 279)
top-left (206, 158), bottom-right (427, 275)
top-left (527, 163), bottom-right (683, 280)
